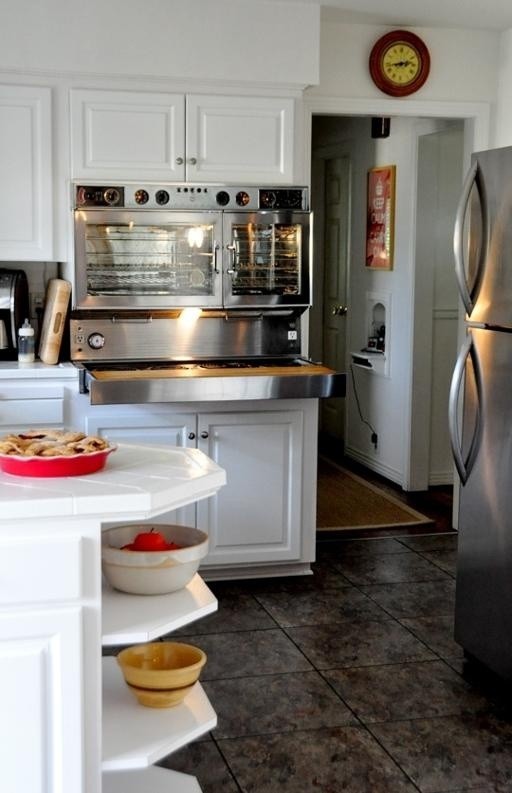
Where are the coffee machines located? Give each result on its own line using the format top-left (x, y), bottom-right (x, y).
top-left (0, 268), bottom-right (29, 361)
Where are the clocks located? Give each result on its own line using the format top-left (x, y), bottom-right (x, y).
top-left (369, 30), bottom-right (430, 97)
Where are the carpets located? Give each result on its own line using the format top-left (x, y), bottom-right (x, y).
top-left (317, 460), bottom-right (432, 530)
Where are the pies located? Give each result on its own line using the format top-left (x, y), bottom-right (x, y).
top-left (0, 430), bottom-right (109, 457)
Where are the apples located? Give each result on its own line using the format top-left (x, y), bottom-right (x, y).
top-left (120, 527), bottom-right (180, 551)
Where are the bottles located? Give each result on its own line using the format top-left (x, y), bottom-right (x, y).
top-left (18, 318), bottom-right (35, 363)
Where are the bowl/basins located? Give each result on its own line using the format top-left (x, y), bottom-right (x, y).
top-left (101, 522), bottom-right (210, 598)
top-left (117, 640), bottom-right (208, 708)
top-left (0, 437), bottom-right (117, 478)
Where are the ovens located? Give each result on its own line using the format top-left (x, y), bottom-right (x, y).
top-left (68, 182), bottom-right (314, 313)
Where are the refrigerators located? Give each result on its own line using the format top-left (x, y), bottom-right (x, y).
top-left (447, 146), bottom-right (512, 688)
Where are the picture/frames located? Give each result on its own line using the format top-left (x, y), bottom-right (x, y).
top-left (364, 165), bottom-right (396, 271)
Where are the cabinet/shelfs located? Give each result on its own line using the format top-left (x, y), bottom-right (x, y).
top-left (69, 82), bottom-right (296, 188)
top-left (0, 359), bottom-right (81, 434)
top-left (83, 395), bottom-right (319, 583)
top-left (0, 82), bottom-right (53, 264)
top-left (99, 484), bottom-right (218, 793)
